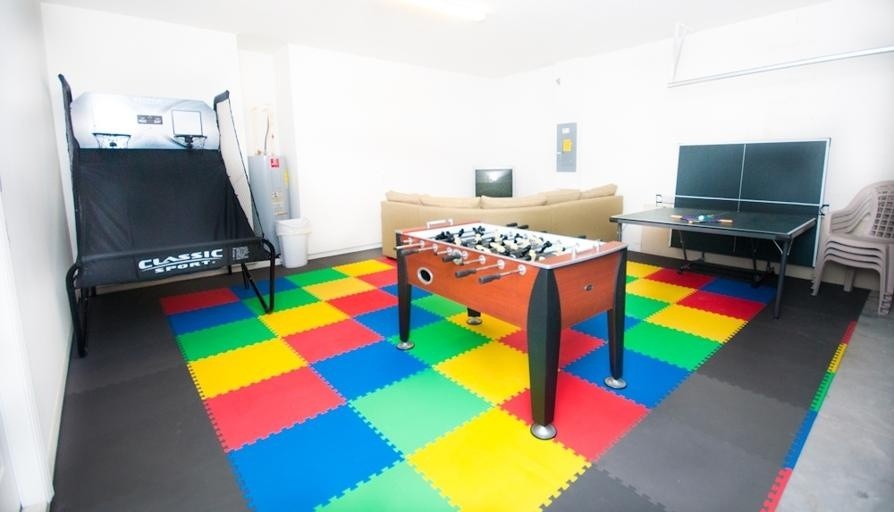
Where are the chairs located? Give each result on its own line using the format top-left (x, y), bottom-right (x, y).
top-left (811, 180), bottom-right (894, 317)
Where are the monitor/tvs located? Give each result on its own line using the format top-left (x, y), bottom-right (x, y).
top-left (475, 169), bottom-right (512, 198)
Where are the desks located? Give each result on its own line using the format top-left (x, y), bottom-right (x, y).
top-left (610, 208), bottom-right (816, 319)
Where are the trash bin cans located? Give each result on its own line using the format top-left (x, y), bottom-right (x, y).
top-left (275, 219), bottom-right (308, 268)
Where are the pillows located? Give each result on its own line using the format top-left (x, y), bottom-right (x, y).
top-left (386, 184), bottom-right (617, 210)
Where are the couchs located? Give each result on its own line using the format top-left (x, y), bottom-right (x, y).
top-left (381, 195), bottom-right (623, 260)
